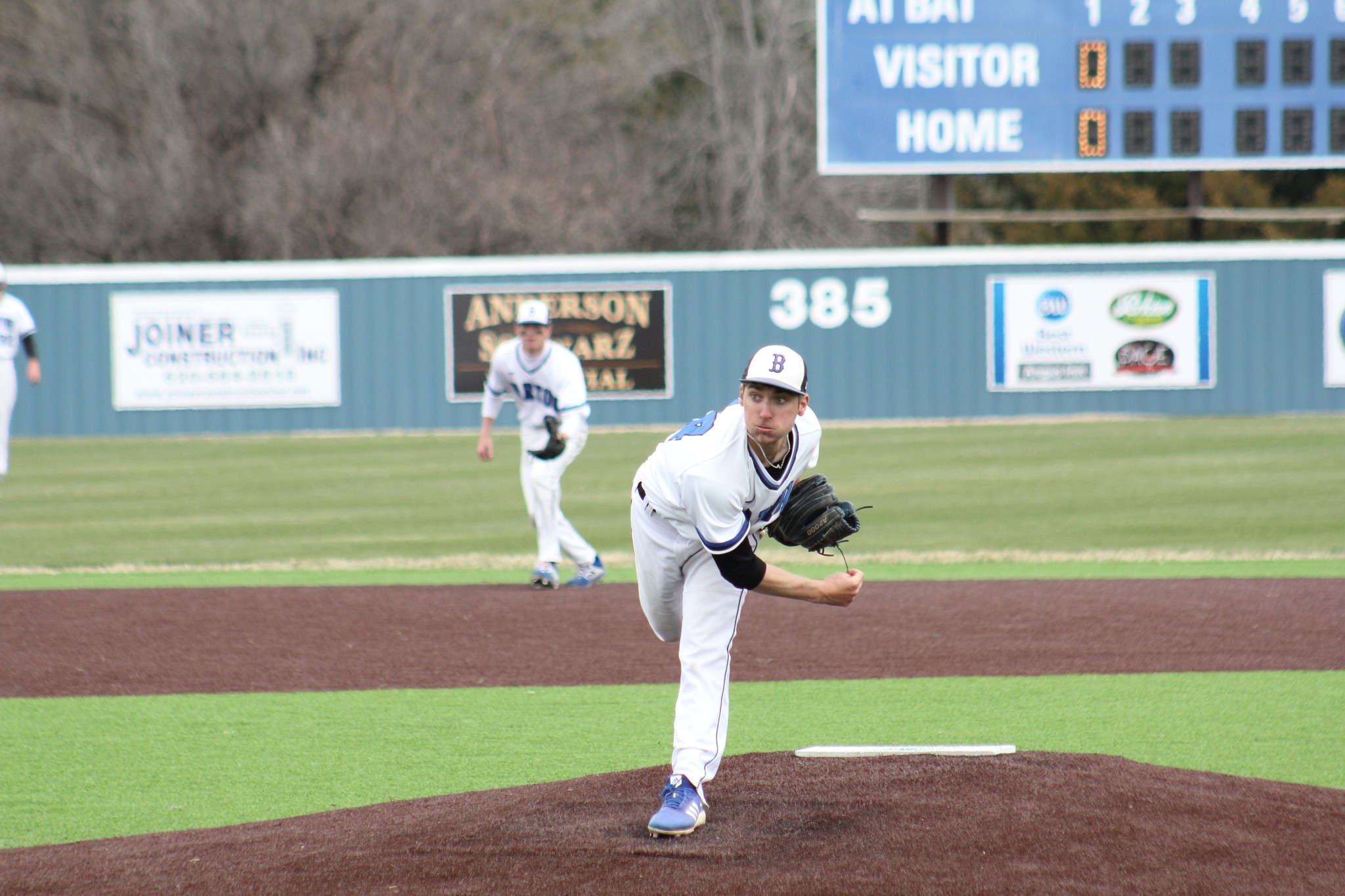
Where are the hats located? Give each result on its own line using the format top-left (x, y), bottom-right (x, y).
top-left (515, 299), bottom-right (549, 326)
top-left (739, 345), bottom-right (808, 396)
top-left (0, 261), bottom-right (7, 283)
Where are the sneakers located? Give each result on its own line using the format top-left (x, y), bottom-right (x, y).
top-left (530, 562), bottom-right (559, 589)
top-left (648, 774), bottom-right (706, 838)
top-left (566, 556), bottom-right (606, 588)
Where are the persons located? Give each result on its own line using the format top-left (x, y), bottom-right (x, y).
top-left (478, 299), bottom-right (604, 590)
top-left (0, 263), bottom-right (41, 474)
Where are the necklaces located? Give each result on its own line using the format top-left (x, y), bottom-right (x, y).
top-left (630, 344), bottom-right (864, 838)
top-left (747, 431), bottom-right (790, 469)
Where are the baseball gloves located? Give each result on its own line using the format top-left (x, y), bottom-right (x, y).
top-left (764, 472), bottom-right (860, 552)
top-left (525, 415), bottom-right (565, 460)
